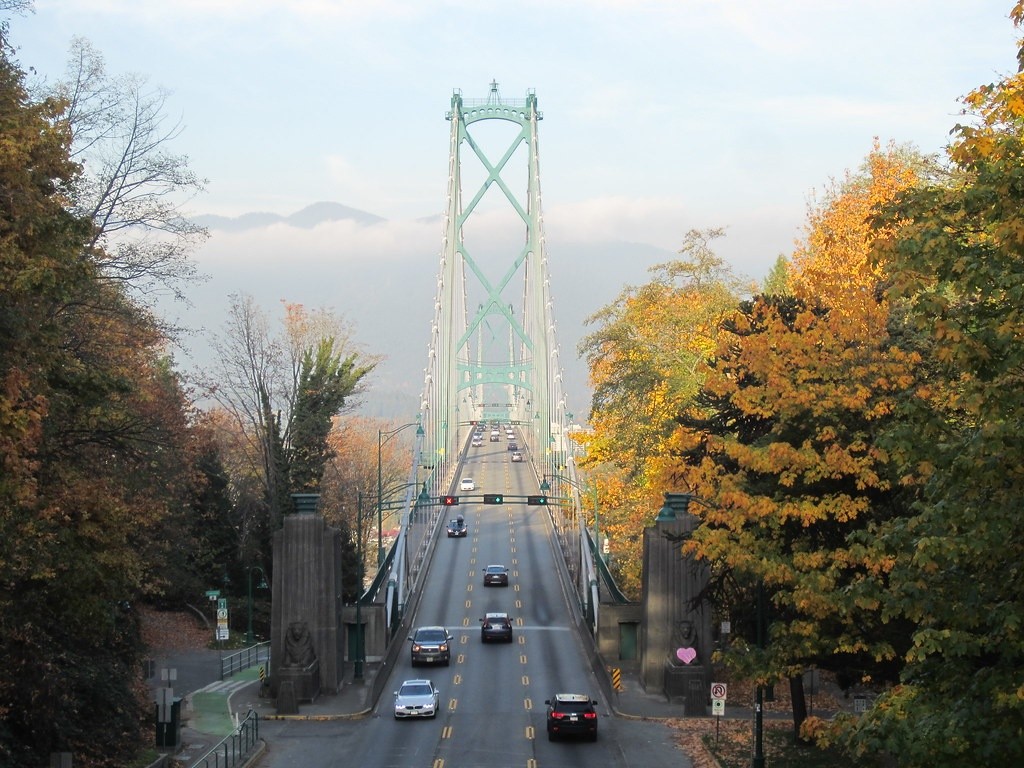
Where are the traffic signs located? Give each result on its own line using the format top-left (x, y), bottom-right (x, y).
top-left (206, 590), bottom-right (229, 641)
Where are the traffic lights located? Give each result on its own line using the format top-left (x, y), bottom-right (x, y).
top-left (492, 404), bottom-right (498, 407)
top-left (528, 496), bottom-right (547, 505)
top-left (506, 404), bottom-right (512, 407)
top-left (484, 494), bottom-right (503, 504)
top-left (444, 496), bottom-right (454, 505)
top-left (491, 421), bottom-right (499, 424)
top-left (478, 404), bottom-right (485, 407)
top-left (511, 421), bottom-right (520, 425)
top-left (470, 421), bottom-right (477, 425)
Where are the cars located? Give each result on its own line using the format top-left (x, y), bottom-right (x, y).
top-left (460, 478), bottom-right (475, 491)
top-left (479, 613), bottom-right (514, 642)
top-left (508, 442), bottom-right (517, 450)
top-left (511, 452), bottom-right (522, 462)
top-left (472, 438), bottom-right (482, 447)
top-left (545, 694), bottom-right (598, 742)
top-left (394, 679), bottom-right (440, 718)
top-left (490, 435), bottom-right (499, 442)
top-left (446, 515), bottom-right (467, 537)
top-left (473, 424), bottom-right (515, 440)
top-left (407, 626), bottom-right (454, 667)
top-left (482, 564), bottom-right (509, 587)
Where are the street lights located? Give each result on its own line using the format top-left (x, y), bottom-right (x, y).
top-left (246, 566), bottom-right (267, 641)
top-left (377, 421), bottom-right (425, 566)
top-left (355, 480), bottom-right (430, 679)
top-left (539, 474), bottom-right (599, 580)
top-left (653, 492), bottom-right (765, 768)
